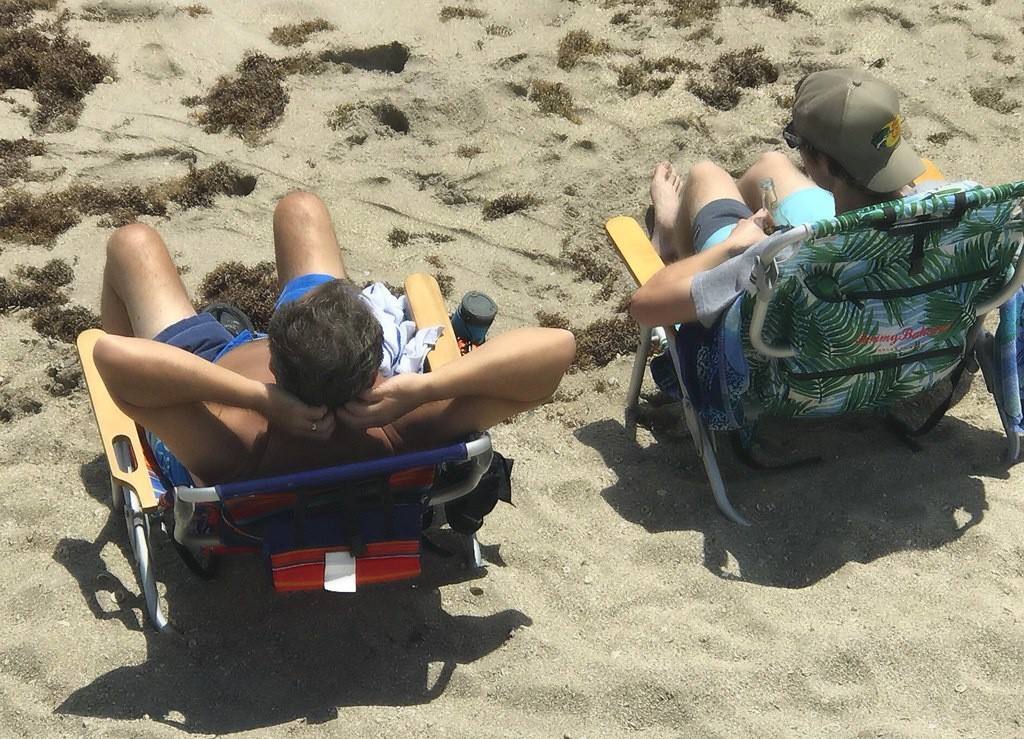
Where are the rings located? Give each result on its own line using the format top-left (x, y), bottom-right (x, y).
top-left (312, 421), bottom-right (317, 432)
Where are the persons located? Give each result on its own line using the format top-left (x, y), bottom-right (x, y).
top-left (91, 189), bottom-right (577, 512)
top-left (629, 67), bottom-right (951, 432)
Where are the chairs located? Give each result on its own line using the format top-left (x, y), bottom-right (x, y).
top-left (77, 273), bottom-right (494, 635)
top-left (605, 158), bottom-right (1022, 526)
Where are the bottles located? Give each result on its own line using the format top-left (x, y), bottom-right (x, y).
top-left (760, 177), bottom-right (793, 263)
top-left (452, 291), bottom-right (499, 345)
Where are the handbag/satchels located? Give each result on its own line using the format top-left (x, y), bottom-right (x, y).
top-left (260, 502), bottom-right (421, 592)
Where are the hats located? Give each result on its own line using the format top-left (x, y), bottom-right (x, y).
top-left (449, 449), bottom-right (516, 508)
top-left (444, 451), bottom-right (500, 537)
top-left (792, 68), bottom-right (927, 194)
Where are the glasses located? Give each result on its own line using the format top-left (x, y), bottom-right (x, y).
top-left (783, 120), bottom-right (811, 149)
